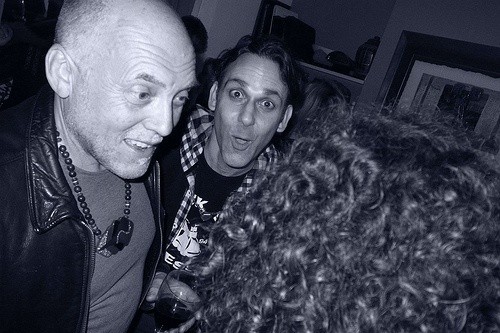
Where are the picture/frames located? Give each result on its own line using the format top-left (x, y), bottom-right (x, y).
top-left (370, 29), bottom-right (500, 142)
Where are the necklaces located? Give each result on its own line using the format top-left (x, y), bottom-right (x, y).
top-left (52, 123), bottom-right (135, 257)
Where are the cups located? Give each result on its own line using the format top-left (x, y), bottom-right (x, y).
top-left (150, 270), bottom-right (207, 333)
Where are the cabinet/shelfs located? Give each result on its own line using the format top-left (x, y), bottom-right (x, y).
top-left (252, 0), bottom-right (366, 87)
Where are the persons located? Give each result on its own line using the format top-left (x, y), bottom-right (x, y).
top-left (1, 1), bottom-right (352, 148)
top-left (137, 34), bottom-right (330, 332)
top-left (0, 0), bottom-right (196, 333)
top-left (187, 91), bottom-right (500, 333)
